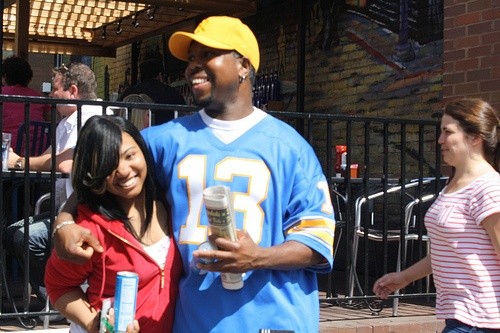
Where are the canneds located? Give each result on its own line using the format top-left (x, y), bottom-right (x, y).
top-left (113, 271), bottom-right (138, 333)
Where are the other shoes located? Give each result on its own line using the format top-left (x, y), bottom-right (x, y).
top-left (38, 306), bottom-right (64, 322)
top-left (16, 295), bottom-right (42, 306)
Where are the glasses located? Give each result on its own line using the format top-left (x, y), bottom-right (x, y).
top-left (56, 63), bottom-right (70, 74)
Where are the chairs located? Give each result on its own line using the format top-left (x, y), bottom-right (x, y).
top-left (16, 121), bottom-right (58, 157)
top-left (347, 178), bottom-right (450, 316)
top-left (33, 93), bottom-right (156, 330)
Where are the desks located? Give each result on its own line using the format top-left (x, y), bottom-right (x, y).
top-left (0, 169), bottom-right (66, 227)
top-left (324, 174), bottom-right (415, 304)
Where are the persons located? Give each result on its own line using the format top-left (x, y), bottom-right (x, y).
top-left (0, 57), bottom-right (53, 156)
top-left (0, 62), bottom-right (115, 321)
top-left (43, 115), bottom-right (183, 333)
top-left (374, 99), bottom-right (500, 333)
top-left (118, 53), bottom-right (186, 125)
top-left (54, 16), bottom-right (336, 333)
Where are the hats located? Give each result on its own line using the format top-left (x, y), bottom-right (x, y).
top-left (168, 15), bottom-right (260, 78)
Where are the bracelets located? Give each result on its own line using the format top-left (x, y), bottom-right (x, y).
top-left (51, 221), bottom-right (74, 237)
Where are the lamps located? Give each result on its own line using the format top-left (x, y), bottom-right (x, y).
top-left (101, 26), bottom-right (107, 39)
top-left (132, 13), bottom-right (140, 27)
top-left (148, 7), bottom-right (156, 20)
top-left (116, 22), bottom-right (122, 35)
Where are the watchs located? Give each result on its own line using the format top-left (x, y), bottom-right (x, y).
top-left (15, 157), bottom-right (24, 169)
